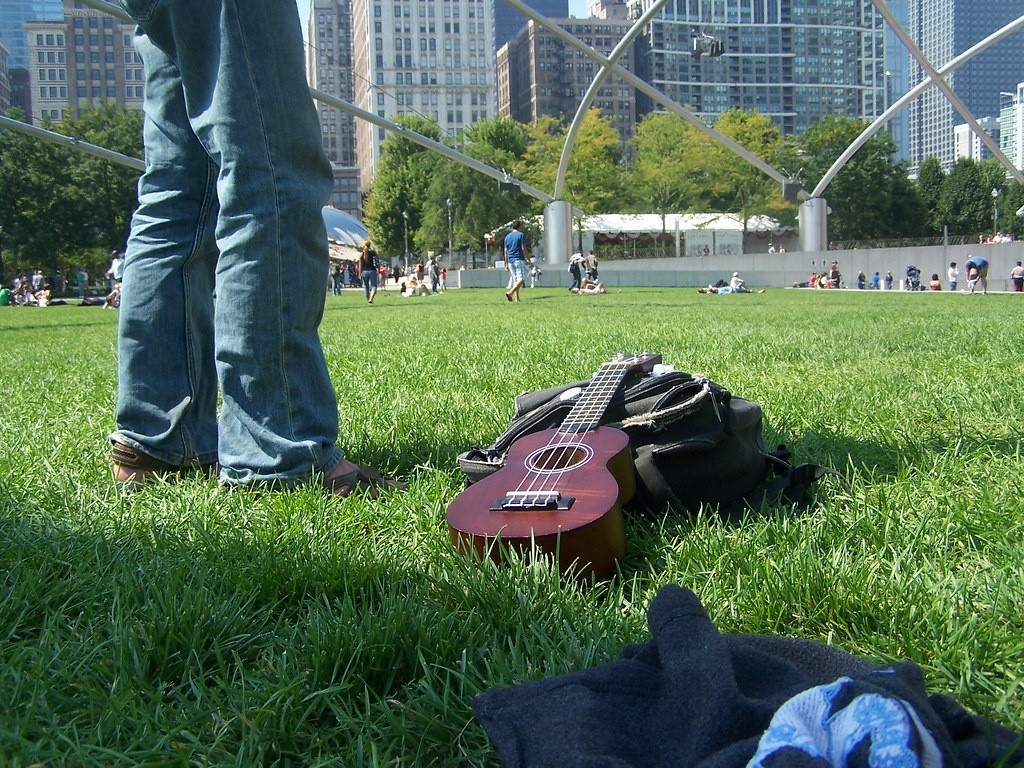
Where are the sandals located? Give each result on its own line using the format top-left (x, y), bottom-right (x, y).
top-left (111, 443), bottom-right (222, 494)
top-left (224, 458), bottom-right (411, 500)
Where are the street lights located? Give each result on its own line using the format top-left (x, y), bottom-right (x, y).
top-left (484, 233), bottom-right (489, 268)
top-left (446, 198), bottom-right (455, 270)
top-left (402, 212), bottom-right (411, 276)
top-left (991, 188), bottom-right (999, 236)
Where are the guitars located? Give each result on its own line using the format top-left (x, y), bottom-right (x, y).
top-left (445, 354), bottom-right (663, 583)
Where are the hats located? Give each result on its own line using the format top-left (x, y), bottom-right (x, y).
top-left (733, 272), bottom-right (739, 277)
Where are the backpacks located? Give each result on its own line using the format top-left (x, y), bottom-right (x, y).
top-left (457, 361), bottom-right (851, 527)
top-left (585, 257), bottom-right (595, 274)
top-left (570, 256), bottom-right (580, 273)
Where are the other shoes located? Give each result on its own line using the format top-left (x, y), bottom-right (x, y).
top-left (568, 288), bottom-right (571, 291)
top-left (506, 292), bottom-right (513, 302)
top-left (749, 289), bottom-right (753, 292)
top-left (368, 298), bottom-right (373, 303)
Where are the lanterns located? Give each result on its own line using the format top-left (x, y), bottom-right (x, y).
top-left (488, 212), bottom-right (799, 257)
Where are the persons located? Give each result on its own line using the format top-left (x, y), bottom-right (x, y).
top-left (871, 272), bottom-right (879, 287)
top-left (77, 268), bottom-right (89, 298)
top-left (376, 262), bottom-right (402, 291)
top-left (568, 249), bottom-right (606, 295)
top-left (327, 259), bottom-right (363, 296)
top-left (947, 257), bottom-right (989, 296)
top-left (698, 271), bottom-right (753, 295)
top-left (703, 244), bottom-right (710, 256)
top-left (503, 220), bottom-right (531, 303)
top-left (357, 240), bottom-right (380, 304)
top-left (1011, 261), bottom-right (1024, 292)
top-left (808, 265), bottom-right (841, 289)
top-left (976, 233), bottom-right (1012, 244)
top-left (767, 242), bottom-right (786, 253)
top-left (886, 271), bottom-right (893, 289)
top-left (102, 0), bottom-right (404, 500)
top-left (0, 268), bottom-right (67, 306)
top-left (857, 271), bottom-right (866, 289)
top-left (929, 274), bottom-right (942, 291)
top-left (78, 250), bottom-right (125, 308)
top-left (400, 251), bottom-right (448, 297)
